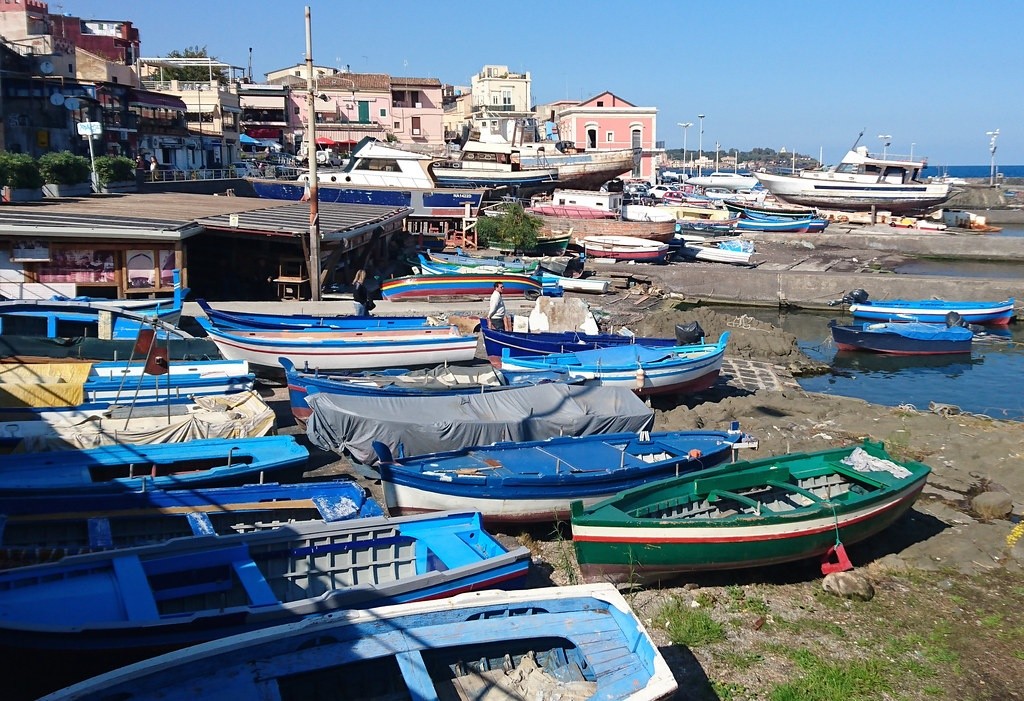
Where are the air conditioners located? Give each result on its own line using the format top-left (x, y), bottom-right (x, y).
top-left (346, 103), bottom-right (352, 109)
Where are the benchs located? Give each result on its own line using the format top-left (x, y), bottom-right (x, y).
top-left (765, 479), bottom-right (823, 502)
top-left (711, 489), bottom-right (772, 513)
top-left (87, 517), bottom-right (114, 549)
top-left (233, 559), bottom-right (276, 608)
top-left (189, 512), bottom-right (215, 536)
top-left (236, 558), bottom-right (275, 606)
top-left (396, 650), bottom-right (440, 700)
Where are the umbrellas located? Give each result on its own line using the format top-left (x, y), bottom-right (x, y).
top-left (240, 133), bottom-right (262, 146)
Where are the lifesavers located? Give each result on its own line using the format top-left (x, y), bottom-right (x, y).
top-left (524, 288), bottom-right (540, 301)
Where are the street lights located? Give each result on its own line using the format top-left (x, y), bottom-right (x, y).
top-left (879, 135), bottom-right (891, 159)
top-left (910, 142), bottom-right (916, 162)
top-left (698, 115), bottom-right (705, 176)
top-left (716, 140), bottom-right (721, 173)
top-left (677, 122), bottom-right (693, 174)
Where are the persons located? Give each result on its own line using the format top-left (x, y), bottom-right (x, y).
top-left (213, 157), bottom-right (221, 174)
top-left (136, 156), bottom-right (144, 168)
top-left (150, 156), bottom-right (159, 182)
top-left (486, 282), bottom-right (505, 330)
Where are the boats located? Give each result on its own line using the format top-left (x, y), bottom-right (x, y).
top-left (880, 207), bottom-right (1004, 234)
top-left (0, 149), bottom-right (834, 478)
top-left (0, 478), bottom-right (386, 556)
top-left (0, 505), bottom-right (533, 659)
top-left (924, 165), bottom-right (1002, 188)
top-left (0, 433), bottom-right (311, 501)
top-left (304, 382), bottom-right (658, 479)
top-left (825, 314), bottom-right (974, 354)
top-left (569, 437), bottom-right (933, 584)
top-left (825, 289), bottom-right (1017, 326)
top-left (750, 125), bottom-right (963, 212)
top-left (372, 420), bottom-right (746, 526)
top-left (30, 580), bottom-right (680, 701)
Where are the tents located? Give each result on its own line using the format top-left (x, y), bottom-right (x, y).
top-left (316, 137), bottom-right (336, 144)
